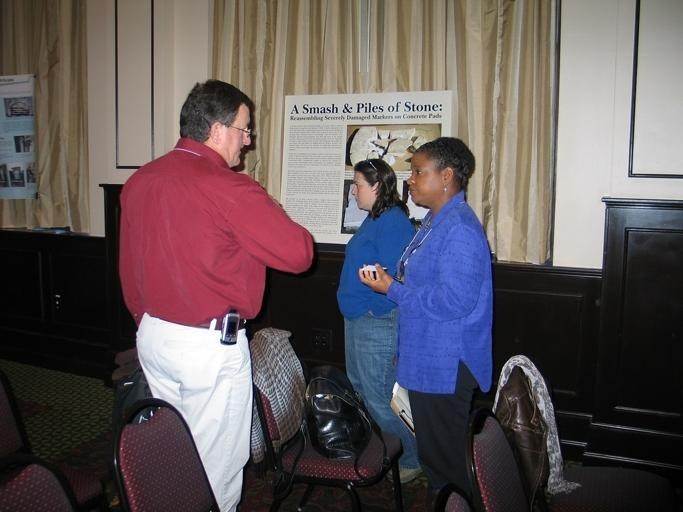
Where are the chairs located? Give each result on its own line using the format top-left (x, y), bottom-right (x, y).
top-left (249, 323), bottom-right (406, 511)
top-left (2, 460), bottom-right (78, 511)
top-left (3, 373), bottom-right (109, 509)
top-left (432, 483), bottom-right (472, 511)
top-left (115, 398), bottom-right (219, 511)
top-left (502, 365), bottom-right (674, 511)
top-left (468, 410), bottom-right (530, 511)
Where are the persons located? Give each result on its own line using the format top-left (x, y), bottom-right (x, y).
top-left (356, 135), bottom-right (495, 511)
top-left (118, 79), bottom-right (315, 512)
top-left (335, 160), bottom-right (421, 485)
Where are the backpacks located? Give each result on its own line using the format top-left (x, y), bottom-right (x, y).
top-left (301, 365), bottom-right (372, 462)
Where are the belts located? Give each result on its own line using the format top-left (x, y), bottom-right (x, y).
top-left (201, 318), bottom-right (246, 331)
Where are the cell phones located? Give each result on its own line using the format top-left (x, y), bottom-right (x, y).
top-left (221, 312), bottom-right (242, 345)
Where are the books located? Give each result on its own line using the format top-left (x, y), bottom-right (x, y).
top-left (387, 378), bottom-right (417, 441)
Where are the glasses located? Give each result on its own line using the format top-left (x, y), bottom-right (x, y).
top-left (219, 121), bottom-right (251, 137)
